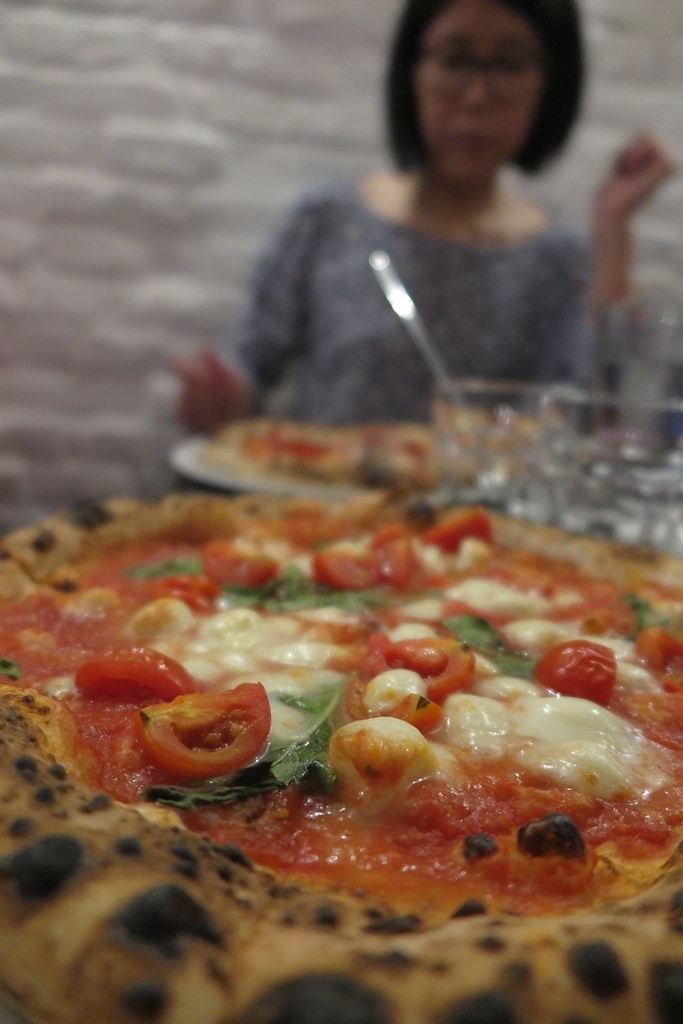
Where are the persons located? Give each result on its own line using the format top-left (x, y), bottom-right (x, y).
top-left (167, 0), bottom-right (675, 437)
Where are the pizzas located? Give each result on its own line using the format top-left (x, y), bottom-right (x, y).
top-left (1, 487), bottom-right (683, 1024)
top-left (203, 417), bottom-right (454, 493)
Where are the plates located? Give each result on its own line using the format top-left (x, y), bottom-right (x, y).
top-left (169, 437), bottom-right (390, 500)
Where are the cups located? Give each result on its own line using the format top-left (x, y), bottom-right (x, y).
top-left (423, 382), bottom-right (682, 558)
